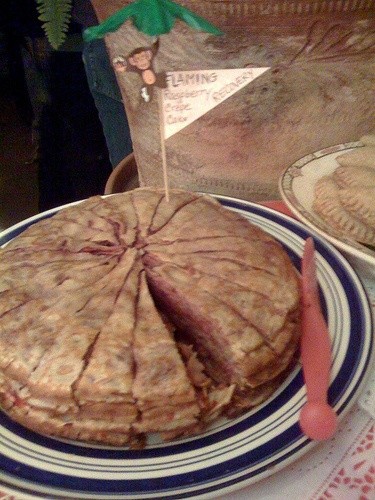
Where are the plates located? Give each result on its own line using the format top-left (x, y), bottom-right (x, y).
top-left (279, 141), bottom-right (374, 268)
top-left (1, 190), bottom-right (373, 500)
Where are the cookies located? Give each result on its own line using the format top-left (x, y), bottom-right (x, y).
top-left (315, 134), bottom-right (375, 248)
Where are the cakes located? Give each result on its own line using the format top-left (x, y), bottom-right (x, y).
top-left (0, 186), bottom-right (303, 446)
